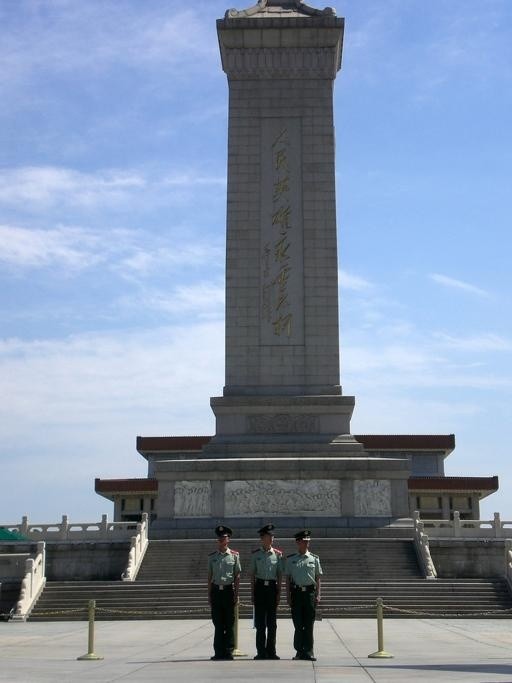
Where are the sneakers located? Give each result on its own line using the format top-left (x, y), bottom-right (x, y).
top-left (210, 652), bottom-right (234, 660)
top-left (292, 652), bottom-right (317, 661)
top-left (253, 653), bottom-right (281, 660)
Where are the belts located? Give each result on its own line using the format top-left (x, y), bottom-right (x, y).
top-left (296, 585), bottom-right (314, 592)
top-left (256, 578), bottom-right (275, 586)
top-left (212, 584), bottom-right (232, 590)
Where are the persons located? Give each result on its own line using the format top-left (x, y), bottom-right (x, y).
top-left (207, 525), bottom-right (242, 660)
top-left (247, 523), bottom-right (284, 659)
top-left (284, 529), bottom-right (323, 660)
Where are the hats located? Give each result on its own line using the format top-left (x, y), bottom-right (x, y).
top-left (294, 530), bottom-right (313, 541)
top-left (214, 526), bottom-right (233, 538)
top-left (257, 525), bottom-right (276, 536)
